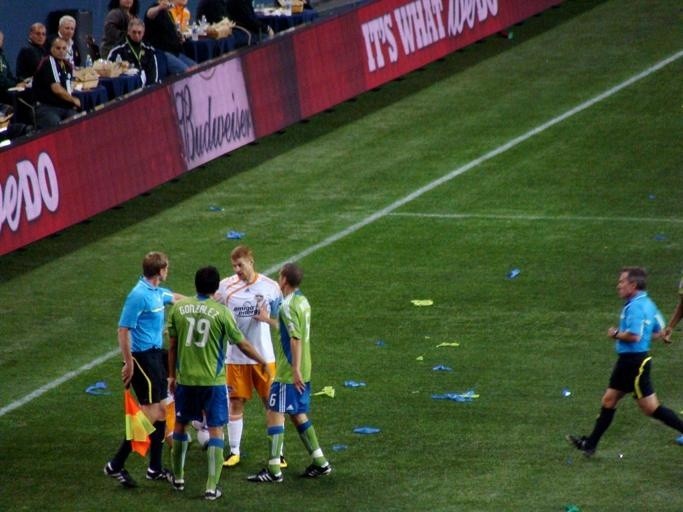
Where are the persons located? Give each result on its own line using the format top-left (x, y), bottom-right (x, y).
top-left (0, 0), bottom-right (192, 142)
top-left (164, 265), bottom-right (270, 501)
top-left (565, 267), bottom-right (683, 457)
top-left (103, 251), bottom-right (187, 488)
top-left (247, 263), bottom-right (331, 483)
top-left (213, 245), bottom-right (287, 468)
top-left (662, 278), bottom-right (683, 444)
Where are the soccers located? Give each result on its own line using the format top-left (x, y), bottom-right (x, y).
top-left (197, 426), bottom-right (224, 446)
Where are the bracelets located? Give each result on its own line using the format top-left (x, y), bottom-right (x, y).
top-left (613, 329), bottom-right (618, 338)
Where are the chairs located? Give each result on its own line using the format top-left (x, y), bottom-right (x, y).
top-left (0, 0), bottom-right (315, 149)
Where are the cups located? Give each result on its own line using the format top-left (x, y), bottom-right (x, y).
top-left (292, 6), bottom-right (302, 13)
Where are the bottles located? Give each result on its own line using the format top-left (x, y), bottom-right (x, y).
top-left (200, 15), bottom-right (207, 37)
top-left (115, 54), bottom-right (122, 64)
top-left (285, 0), bottom-right (291, 16)
top-left (85, 55), bottom-right (92, 69)
top-left (190, 22), bottom-right (198, 42)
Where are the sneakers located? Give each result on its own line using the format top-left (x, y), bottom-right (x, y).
top-left (570, 435), bottom-right (595, 458)
top-left (246, 457), bottom-right (287, 483)
top-left (223, 452), bottom-right (240, 466)
top-left (300, 462), bottom-right (332, 478)
top-left (205, 488), bottom-right (222, 500)
top-left (103, 461), bottom-right (185, 491)
